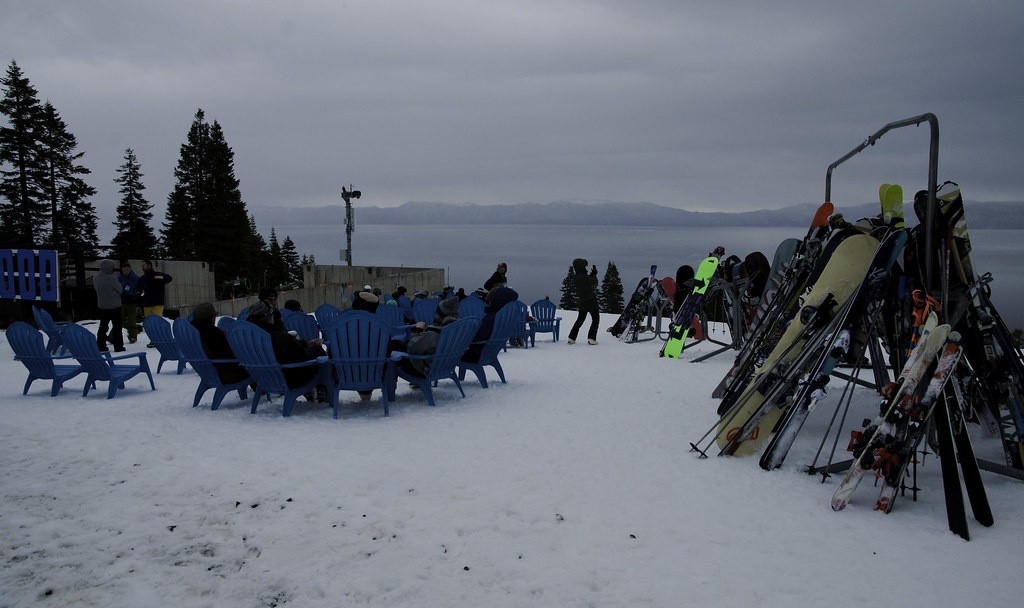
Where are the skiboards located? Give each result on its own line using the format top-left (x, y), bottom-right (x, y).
top-left (830, 310), bottom-right (964, 514)
top-left (624, 264), bottom-right (658, 344)
top-left (716, 227), bottom-right (916, 471)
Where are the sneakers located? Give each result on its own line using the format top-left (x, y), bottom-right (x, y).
top-left (568, 338), bottom-right (576, 344)
top-left (587, 338), bottom-right (598, 345)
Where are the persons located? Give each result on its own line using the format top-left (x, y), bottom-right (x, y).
top-left (381, 297), bottom-right (457, 388)
top-left (567, 258), bottom-right (600, 345)
top-left (93, 259), bottom-right (173, 353)
top-left (191, 287), bottom-right (391, 404)
top-left (354, 262), bottom-right (538, 347)
top-left (461, 287), bottom-right (518, 364)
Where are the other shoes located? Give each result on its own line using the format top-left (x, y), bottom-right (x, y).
top-left (115, 346), bottom-right (126, 352)
top-left (147, 342), bottom-right (155, 347)
top-left (100, 346), bottom-right (109, 351)
top-left (130, 337), bottom-right (135, 343)
top-left (517, 337), bottom-right (524, 346)
top-left (511, 340), bottom-right (520, 347)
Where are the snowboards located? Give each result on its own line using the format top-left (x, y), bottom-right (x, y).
top-left (611, 277), bottom-right (649, 336)
top-left (674, 264), bottom-right (694, 315)
top-left (664, 256), bottom-right (719, 359)
top-left (712, 229), bottom-right (866, 400)
top-left (716, 234), bottom-right (881, 456)
top-left (662, 277), bottom-right (707, 340)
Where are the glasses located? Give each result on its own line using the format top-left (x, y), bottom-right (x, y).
top-left (267, 297), bottom-right (277, 301)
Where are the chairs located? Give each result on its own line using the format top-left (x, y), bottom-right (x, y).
top-left (5, 290), bottom-right (563, 418)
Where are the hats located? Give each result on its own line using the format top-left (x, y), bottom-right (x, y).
top-left (485, 287), bottom-right (500, 301)
top-left (364, 285), bottom-right (372, 289)
top-left (285, 299), bottom-right (303, 312)
top-left (386, 299), bottom-right (397, 306)
top-left (442, 286), bottom-right (454, 294)
top-left (258, 287), bottom-right (277, 300)
top-left (436, 296), bottom-right (459, 318)
top-left (249, 301), bottom-right (274, 319)
top-left (192, 303), bottom-right (218, 320)
top-left (414, 289), bottom-right (429, 299)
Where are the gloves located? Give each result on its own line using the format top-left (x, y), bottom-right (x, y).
top-left (592, 266), bottom-right (597, 275)
